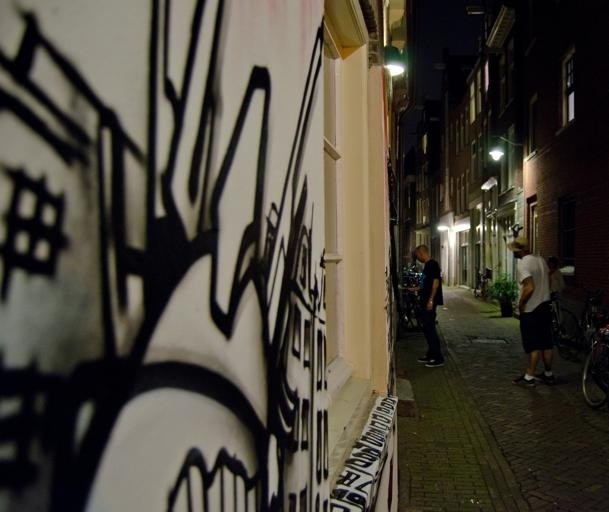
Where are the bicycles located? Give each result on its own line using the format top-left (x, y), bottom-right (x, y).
top-left (549, 290), bottom-right (609, 407)
top-left (474, 273), bottom-right (489, 300)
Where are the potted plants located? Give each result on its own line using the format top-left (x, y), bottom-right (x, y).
top-left (482, 271), bottom-right (520, 318)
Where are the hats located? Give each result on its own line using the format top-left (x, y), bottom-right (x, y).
top-left (505, 236), bottom-right (531, 252)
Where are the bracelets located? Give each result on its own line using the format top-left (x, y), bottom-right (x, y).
top-left (428, 299), bottom-right (433, 303)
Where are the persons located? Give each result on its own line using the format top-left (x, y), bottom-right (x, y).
top-left (406, 243), bottom-right (445, 369)
top-left (505, 237), bottom-right (559, 388)
top-left (546, 255), bottom-right (564, 300)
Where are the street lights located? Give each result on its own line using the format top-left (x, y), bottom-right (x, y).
top-left (436, 224), bottom-right (452, 287)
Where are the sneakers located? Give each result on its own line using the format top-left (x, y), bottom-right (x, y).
top-left (417, 356), bottom-right (430, 363)
top-left (534, 371), bottom-right (555, 385)
top-left (424, 358), bottom-right (445, 368)
top-left (511, 377), bottom-right (537, 388)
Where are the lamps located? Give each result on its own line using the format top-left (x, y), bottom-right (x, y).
top-left (487, 131), bottom-right (524, 163)
